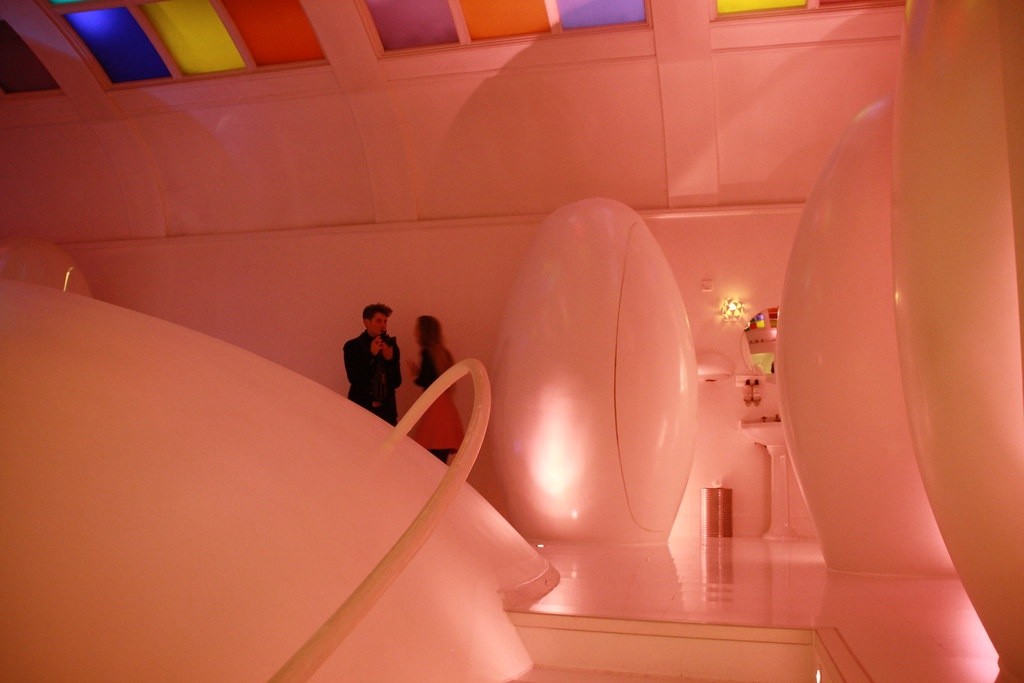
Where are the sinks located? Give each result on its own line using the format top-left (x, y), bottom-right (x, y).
top-left (739, 419), bottom-right (787, 446)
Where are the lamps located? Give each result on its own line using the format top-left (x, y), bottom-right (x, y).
top-left (721, 297), bottom-right (744, 321)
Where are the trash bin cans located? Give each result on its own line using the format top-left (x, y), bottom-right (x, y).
top-left (701, 487), bottom-right (733, 538)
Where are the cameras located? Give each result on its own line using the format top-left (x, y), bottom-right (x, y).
top-left (379, 329), bottom-right (396, 348)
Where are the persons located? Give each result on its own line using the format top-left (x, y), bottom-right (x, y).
top-left (402, 314), bottom-right (465, 465)
top-left (342, 303), bottom-right (403, 429)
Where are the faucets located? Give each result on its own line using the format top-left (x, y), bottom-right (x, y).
top-left (774, 414), bottom-right (781, 422)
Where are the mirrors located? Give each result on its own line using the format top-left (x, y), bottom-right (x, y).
top-left (740, 308), bottom-right (779, 376)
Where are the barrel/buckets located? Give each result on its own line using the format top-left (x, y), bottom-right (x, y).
top-left (701, 487), bottom-right (734, 539)
